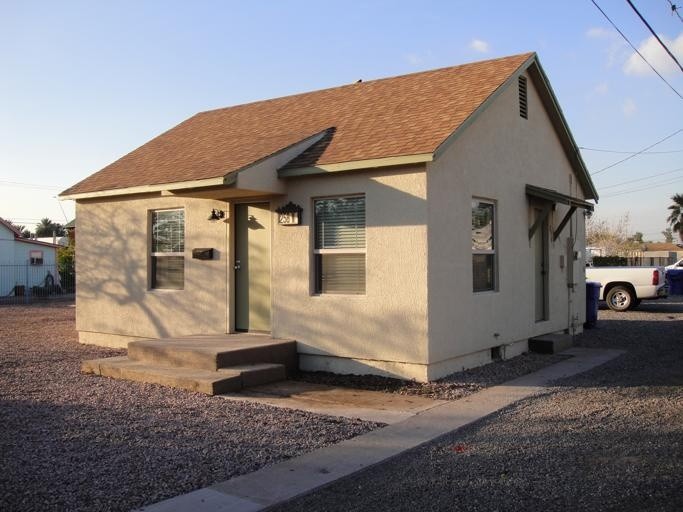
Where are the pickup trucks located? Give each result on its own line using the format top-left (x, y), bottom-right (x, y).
top-left (585, 265), bottom-right (666, 312)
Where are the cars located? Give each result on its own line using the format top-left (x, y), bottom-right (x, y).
top-left (665, 258), bottom-right (683, 271)
top-left (665, 269), bottom-right (683, 296)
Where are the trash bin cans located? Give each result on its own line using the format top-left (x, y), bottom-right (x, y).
top-left (585, 282), bottom-right (603, 326)
top-left (668, 270), bottom-right (683, 296)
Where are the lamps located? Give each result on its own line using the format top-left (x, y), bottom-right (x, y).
top-left (207, 208), bottom-right (224, 224)
